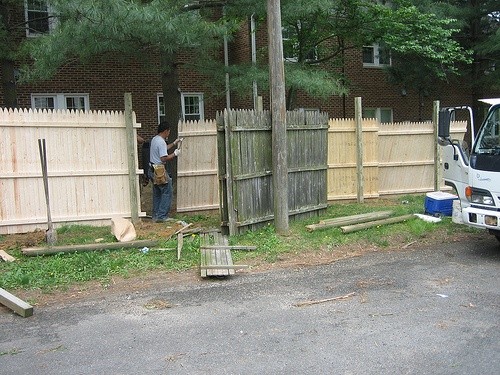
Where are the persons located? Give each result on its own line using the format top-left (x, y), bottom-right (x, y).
top-left (147, 122), bottom-right (182, 224)
top-left (136, 134), bottom-right (149, 196)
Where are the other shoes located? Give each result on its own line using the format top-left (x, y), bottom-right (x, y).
top-left (153, 218), bottom-right (175, 223)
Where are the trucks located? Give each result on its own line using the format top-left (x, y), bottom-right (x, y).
top-left (439, 97), bottom-right (500, 244)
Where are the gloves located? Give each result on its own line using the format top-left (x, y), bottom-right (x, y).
top-left (173, 138), bottom-right (180, 145)
top-left (174, 148), bottom-right (181, 156)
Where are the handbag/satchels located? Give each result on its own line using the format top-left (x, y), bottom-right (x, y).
top-left (152, 165), bottom-right (169, 186)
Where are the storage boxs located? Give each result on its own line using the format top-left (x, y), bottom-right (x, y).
top-left (424, 191), bottom-right (458, 217)
